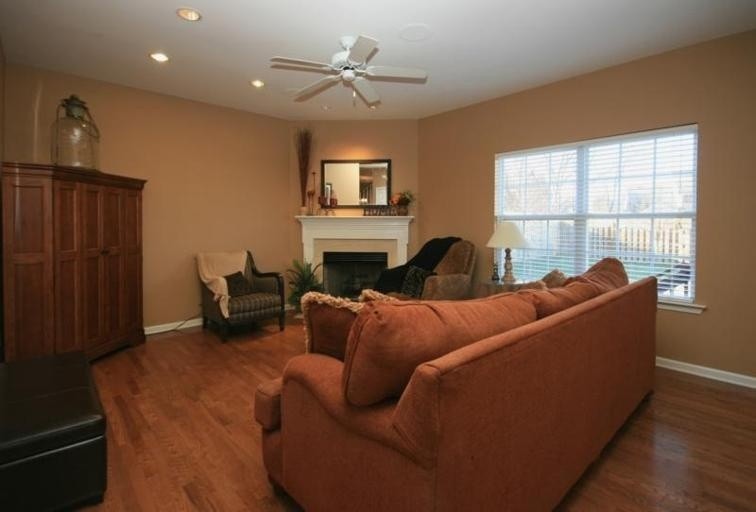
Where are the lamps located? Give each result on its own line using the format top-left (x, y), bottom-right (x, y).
top-left (485, 221), bottom-right (535, 280)
top-left (175, 7), bottom-right (203, 24)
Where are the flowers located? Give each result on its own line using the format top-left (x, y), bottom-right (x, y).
top-left (389, 191), bottom-right (416, 205)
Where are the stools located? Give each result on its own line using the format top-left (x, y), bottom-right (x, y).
top-left (1, 349), bottom-right (107, 512)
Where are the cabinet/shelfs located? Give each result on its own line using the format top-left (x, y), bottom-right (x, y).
top-left (1, 159), bottom-right (148, 365)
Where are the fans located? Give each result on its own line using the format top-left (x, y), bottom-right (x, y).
top-left (267, 33), bottom-right (428, 105)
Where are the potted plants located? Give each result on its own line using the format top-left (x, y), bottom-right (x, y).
top-left (296, 128), bottom-right (312, 215)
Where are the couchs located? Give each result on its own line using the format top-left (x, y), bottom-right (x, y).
top-left (254, 258), bottom-right (659, 512)
top-left (377, 237), bottom-right (477, 302)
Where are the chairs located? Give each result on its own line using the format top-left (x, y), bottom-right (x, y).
top-left (194, 249), bottom-right (285, 344)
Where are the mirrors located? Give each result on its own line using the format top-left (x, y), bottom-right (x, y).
top-left (319, 158), bottom-right (392, 209)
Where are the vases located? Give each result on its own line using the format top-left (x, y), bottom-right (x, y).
top-left (397, 205), bottom-right (408, 216)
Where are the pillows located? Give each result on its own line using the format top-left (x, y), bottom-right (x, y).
top-left (517, 280), bottom-right (600, 319)
top-left (561, 256), bottom-right (630, 296)
top-left (341, 291), bottom-right (538, 408)
